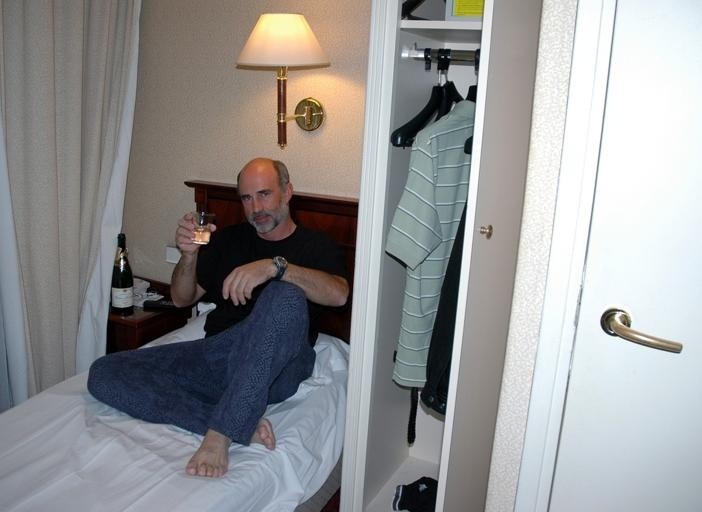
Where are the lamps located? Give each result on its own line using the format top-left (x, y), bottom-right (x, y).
top-left (234, 13), bottom-right (331, 148)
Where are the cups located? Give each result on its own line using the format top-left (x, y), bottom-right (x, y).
top-left (190, 210), bottom-right (217, 245)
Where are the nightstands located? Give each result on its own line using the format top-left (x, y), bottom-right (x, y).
top-left (106, 275), bottom-right (192, 356)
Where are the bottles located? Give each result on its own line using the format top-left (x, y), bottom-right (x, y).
top-left (111, 233), bottom-right (134, 317)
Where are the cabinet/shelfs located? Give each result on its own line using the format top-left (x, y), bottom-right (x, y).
top-left (338, 0), bottom-right (544, 512)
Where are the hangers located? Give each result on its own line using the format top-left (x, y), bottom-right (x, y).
top-left (391, 48), bottom-right (480, 154)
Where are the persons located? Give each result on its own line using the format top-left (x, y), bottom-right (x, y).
top-left (87, 159), bottom-right (355, 477)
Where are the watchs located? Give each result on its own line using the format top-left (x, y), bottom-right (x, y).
top-left (272, 254), bottom-right (288, 281)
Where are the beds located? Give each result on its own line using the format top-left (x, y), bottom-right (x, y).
top-left (0, 180), bottom-right (358, 512)
